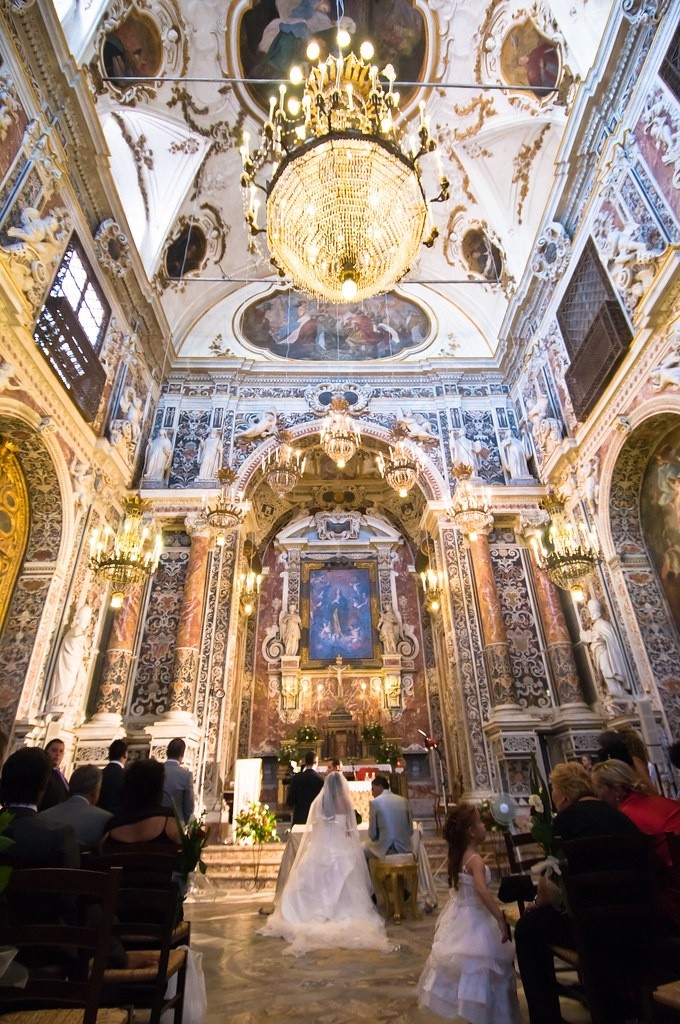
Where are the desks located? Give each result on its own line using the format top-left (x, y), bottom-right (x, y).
top-left (295, 763), bottom-right (392, 779)
top-left (275, 820), bottom-right (438, 913)
top-left (347, 779), bottom-right (392, 824)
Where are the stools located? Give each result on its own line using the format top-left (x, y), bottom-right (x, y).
top-left (370, 857), bottom-right (424, 925)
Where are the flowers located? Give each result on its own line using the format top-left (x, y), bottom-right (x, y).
top-left (527, 794), bottom-right (545, 814)
top-left (360, 721), bottom-right (396, 763)
top-left (475, 794), bottom-right (506, 832)
top-left (233, 800), bottom-right (282, 844)
top-left (277, 724), bottom-right (318, 763)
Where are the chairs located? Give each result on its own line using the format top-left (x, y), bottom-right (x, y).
top-left (505, 829), bottom-right (680, 1024)
top-left (0, 842), bottom-right (191, 1024)
top-left (432, 782), bottom-right (462, 835)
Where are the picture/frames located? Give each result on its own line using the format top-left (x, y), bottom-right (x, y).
top-left (298, 560), bottom-right (382, 670)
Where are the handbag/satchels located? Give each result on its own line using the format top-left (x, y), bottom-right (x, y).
top-left (160, 945), bottom-right (206, 1024)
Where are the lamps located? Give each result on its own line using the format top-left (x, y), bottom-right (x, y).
top-left (259, 430), bottom-right (308, 502)
top-left (319, 398), bottom-right (363, 468)
top-left (419, 570), bottom-right (443, 614)
top-left (200, 466), bottom-right (254, 547)
top-left (514, 488), bottom-right (605, 602)
top-left (237, 29), bottom-right (429, 306)
top-left (237, 571), bottom-right (264, 618)
top-left (447, 462), bottom-right (495, 542)
top-left (373, 420), bottom-right (426, 499)
top-left (85, 488), bottom-right (163, 609)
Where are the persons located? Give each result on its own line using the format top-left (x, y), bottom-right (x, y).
top-left (398, 409), bottom-right (439, 440)
top-left (234, 410), bottom-right (276, 438)
top-left (514, 731), bottom-right (680, 1024)
top-left (449, 428), bottom-right (480, 477)
top-left (124, 397), bottom-right (143, 443)
top-left (579, 600), bottom-right (632, 696)
top-left (287, 752), bottom-right (325, 825)
top-left (585, 464), bottom-right (599, 513)
top-left (7, 217), bottom-right (60, 245)
top-left (362, 777), bottom-right (413, 905)
top-left (197, 429), bottom-right (223, 479)
top-left (71, 460), bottom-right (87, 512)
top-left (282, 605), bottom-right (301, 656)
top-left (326, 758), bottom-right (342, 774)
top-left (144, 428), bottom-right (172, 481)
top-left (49, 605), bottom-right (92, 710)
top-left (0, 738), bottom-right (195, 967)
top-left (606, 232), bottom-right (653, 263)
top-left (416, 803), bottom-right (519, 1024)
top-left (648, 355), bottom-right (680, 394)
top-left (499, 429), bottom-right (533, 477)
top-left (256, 771), bottom-right (397, 954)
top-left (377, 605), bottom-right (398, 653)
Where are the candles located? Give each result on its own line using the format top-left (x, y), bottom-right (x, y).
top-left (282, 677), bottom-right (391, 714)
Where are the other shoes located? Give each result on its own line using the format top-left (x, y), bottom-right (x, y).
top-left (403, 889), bottom-right (411, 902)
top-left (371, 893), bottom-right (376, 905)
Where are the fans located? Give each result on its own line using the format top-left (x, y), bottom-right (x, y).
top-left (493, 792), bottom-right (539, 885)
